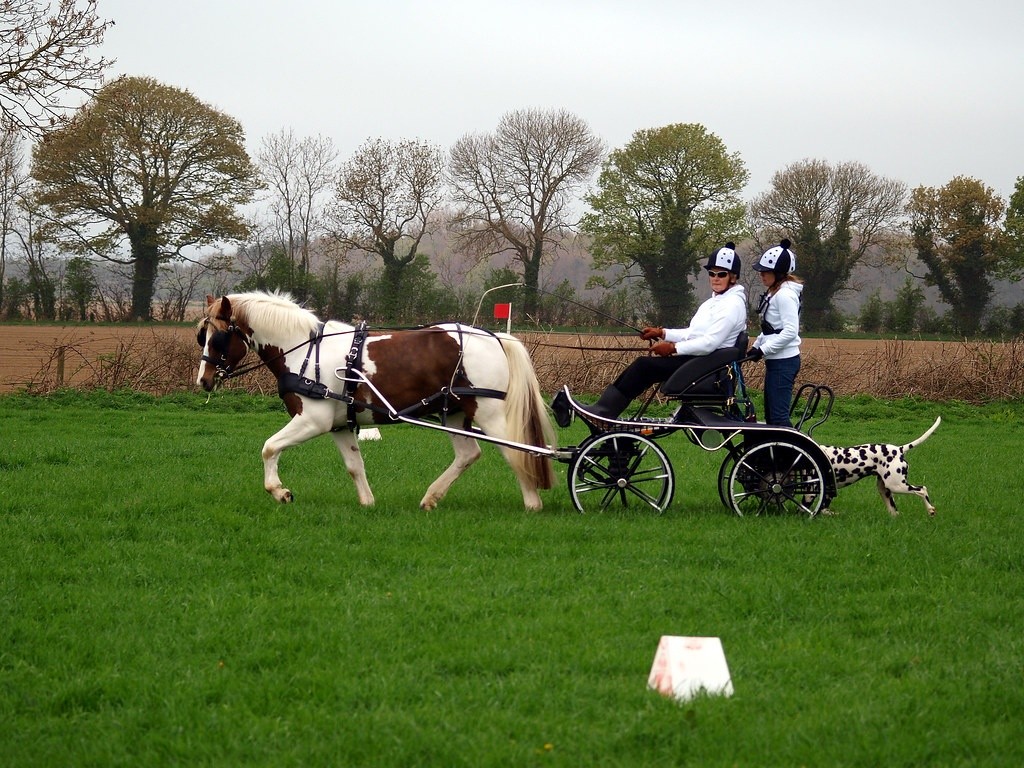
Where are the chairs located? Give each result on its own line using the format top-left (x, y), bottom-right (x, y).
top-left (660, 330), bottom-right (748, 404)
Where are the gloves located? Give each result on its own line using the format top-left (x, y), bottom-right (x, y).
top-left (639, 326), bottom-right (662, 340)
top-left (649, 341), bottom-right (675, 355)
top-left (746, 347), bottom-right (763, 362)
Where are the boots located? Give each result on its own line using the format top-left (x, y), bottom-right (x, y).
top-left (572, 382), bottom-right (631, 420)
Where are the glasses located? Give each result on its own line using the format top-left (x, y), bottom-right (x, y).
top-left (708, 269), bottom-right (729, 278)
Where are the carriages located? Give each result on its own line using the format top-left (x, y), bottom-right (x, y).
top-left (196, 286), bottom-right (835, 521)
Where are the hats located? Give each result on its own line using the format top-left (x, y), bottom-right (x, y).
top-left (703, 241), bottom-right (740, 279)
top-left (752, 238), bottom-right (795, 272)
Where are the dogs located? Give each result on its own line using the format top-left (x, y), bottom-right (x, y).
top-left (795, 416), bottom-right (943, 519)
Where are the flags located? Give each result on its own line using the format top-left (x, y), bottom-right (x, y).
top-left (493, 304), bottom-right (509, 318)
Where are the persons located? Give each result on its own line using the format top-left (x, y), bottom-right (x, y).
top-left (746, 238), bottom-right (804, 426)
top-left (574, 240), bottom-right (747, 427)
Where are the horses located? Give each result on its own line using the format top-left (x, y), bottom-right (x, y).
top-left (194, 285), bottom-right (558, 513)
